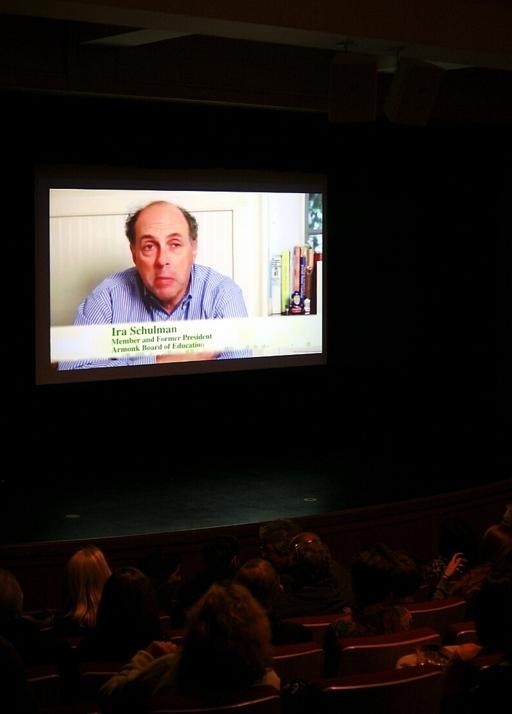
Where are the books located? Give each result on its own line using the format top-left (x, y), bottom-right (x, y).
top-left (265, 241), bottom-right (322, 316)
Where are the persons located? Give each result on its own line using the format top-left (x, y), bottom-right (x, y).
top-left (57, 199), bottom-right (257, 371)
top-left (0, 503), bottom-right (512, 714)
